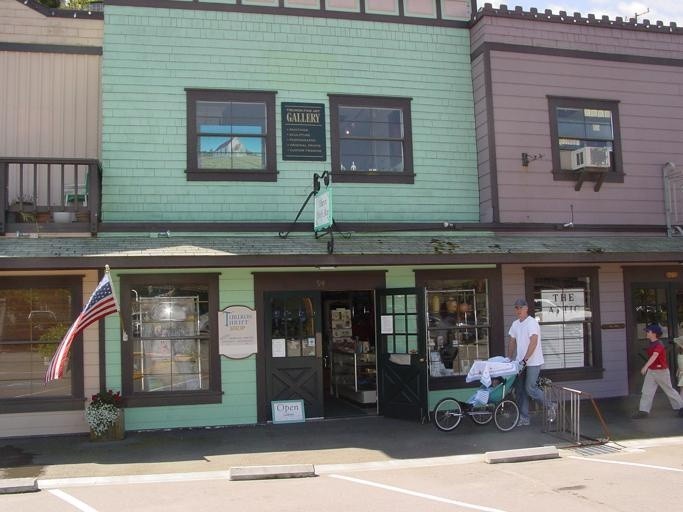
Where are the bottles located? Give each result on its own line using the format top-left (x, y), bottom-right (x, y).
top-left (432, 294), bottom-right (439, 314)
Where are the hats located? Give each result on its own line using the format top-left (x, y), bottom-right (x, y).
top-left (674, 336), bottom-right (683, 349)
top-left (643, 324), bottom-right (662, 336)
top-left (512, 299), bottom-right (528, 306)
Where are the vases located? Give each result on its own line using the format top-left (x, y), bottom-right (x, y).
top-left (6, 211), bottom-right (16, 223)
top-left (90, 408), bottom-right (124, 440)
top-left (37, 205), bottom-right (90, 223)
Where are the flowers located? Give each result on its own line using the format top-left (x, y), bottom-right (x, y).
top-left (86, 390), bottom-right (124, 437)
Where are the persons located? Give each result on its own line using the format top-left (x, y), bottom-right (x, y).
top-left (506, 299), bottom-right (558, 426)
top-left (631, 323), bottom-right (683, 422)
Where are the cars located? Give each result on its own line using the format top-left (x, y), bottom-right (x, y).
top-left (28, 310), bottom-right (58, 340)
top-left (144, 303), bottom-right (208, 337)
top-left (131, 361), bottom-right (196, 393)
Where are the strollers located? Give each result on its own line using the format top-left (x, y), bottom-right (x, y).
top-left (433, 356), bottom-right (521, 432)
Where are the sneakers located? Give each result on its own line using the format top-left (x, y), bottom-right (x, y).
top-left (513, 416), bottom-right (530, 427)
top-left (679, 407), bottom-right (683, 416)
top-left (547, 402), bottom-right (558, 422)
top-left (632, 411), bottom-right (649, 418)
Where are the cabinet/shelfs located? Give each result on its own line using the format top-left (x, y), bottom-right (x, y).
top-left (425, 286), bottom-right (478, 376)
top-left (325, 300), bottom-right (376, 404)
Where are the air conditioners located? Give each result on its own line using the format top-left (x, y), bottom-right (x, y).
top-left (570, 147), bottom-right (610, 170)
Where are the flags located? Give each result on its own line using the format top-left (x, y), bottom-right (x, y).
top-left (43, 274), bottom-right (119, 387)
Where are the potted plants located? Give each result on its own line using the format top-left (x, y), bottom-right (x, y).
top-left (15, 193), bottom-right (33, 212)
